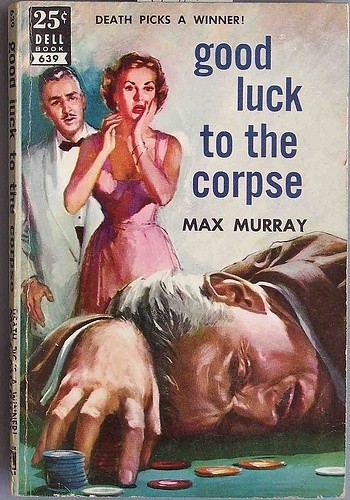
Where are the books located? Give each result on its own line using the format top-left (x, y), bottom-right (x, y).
top-left (6, 1), bottom-right (350, 500)
